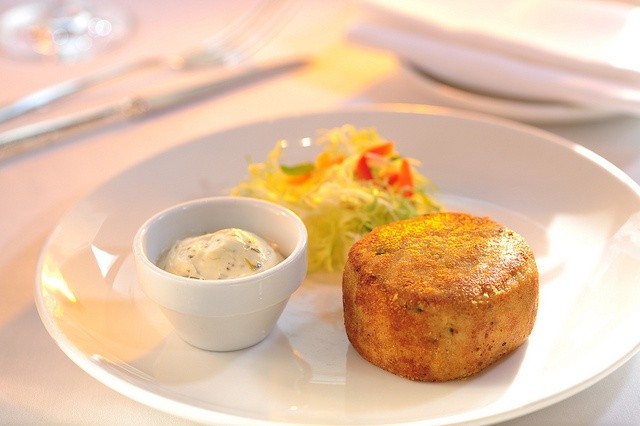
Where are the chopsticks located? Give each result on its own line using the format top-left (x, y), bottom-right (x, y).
top-left (0, 38), bottom-right (315, 147)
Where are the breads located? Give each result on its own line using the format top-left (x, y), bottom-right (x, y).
top-left (342, 213), bottom-right (539, 381)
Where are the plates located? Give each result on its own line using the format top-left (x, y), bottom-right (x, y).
top-left (394, 44), bottom-right (640, 124)
top-left (32, 107), bottom-right (640, 425)
